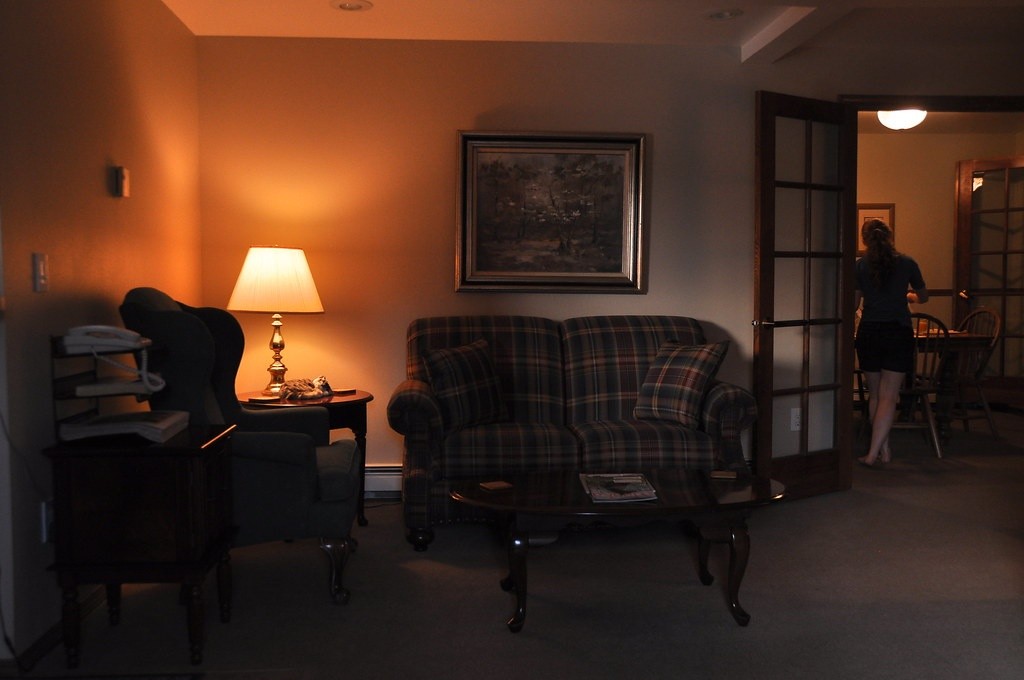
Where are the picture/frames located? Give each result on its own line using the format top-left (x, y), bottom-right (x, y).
top-left (455, 129), bottom-right (646, 295)
top-left (856, 202), bottom-right (895, 256)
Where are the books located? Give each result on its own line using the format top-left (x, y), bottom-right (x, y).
top-left (578, 471), bottom-right (659, 504)
top-left (60, 410), bottom-right (192, 443)
top-left (75, 373), bottom-right (162, 395)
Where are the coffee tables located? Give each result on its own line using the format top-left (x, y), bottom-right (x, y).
top-left (448, 464), bottom-right (785, 632)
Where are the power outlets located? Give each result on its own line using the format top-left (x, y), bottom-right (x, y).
top-left (791, 408), bottom-right (801, 431)
top-left (41, 497), bottom-right (56, 545)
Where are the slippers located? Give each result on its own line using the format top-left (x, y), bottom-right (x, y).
top-left (877, 453), bottom-right (890, 465)
top-left (858, 455), bottom-right (873, 467)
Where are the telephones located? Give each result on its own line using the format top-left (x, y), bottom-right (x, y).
top-left (58, 324), bottom-right (152, 357)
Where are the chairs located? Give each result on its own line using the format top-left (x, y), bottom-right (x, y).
top-left (916, 308), bottom-right (1001, 444)
top-left (854, 313), bottom-right (951, 459)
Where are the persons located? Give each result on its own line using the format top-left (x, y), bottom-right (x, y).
top-left (854, 218), bottom-right (928, 466)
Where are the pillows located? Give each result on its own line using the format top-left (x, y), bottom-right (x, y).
top-left (419, 339), bottom-right (510, 435)
top-left (631, 338), bottom-right (731, 428)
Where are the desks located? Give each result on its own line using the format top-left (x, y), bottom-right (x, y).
top-left (238, 386), bottom-right (374, 526)
top-left (851, 333), bottom-right (994, 431)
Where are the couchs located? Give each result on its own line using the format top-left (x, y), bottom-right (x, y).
top-left (119, 288), bottom-right (361, 606)
top-left (386, 314), bottom-right (758, 551)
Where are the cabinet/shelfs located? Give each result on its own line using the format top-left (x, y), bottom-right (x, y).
top-left (51, 423), bottom-right (237, 668)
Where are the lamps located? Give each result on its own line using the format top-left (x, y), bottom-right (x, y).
top-left (876, 109), bottom-right (927, 130)
top-left (226, 247), bottom-right (325, 396)
